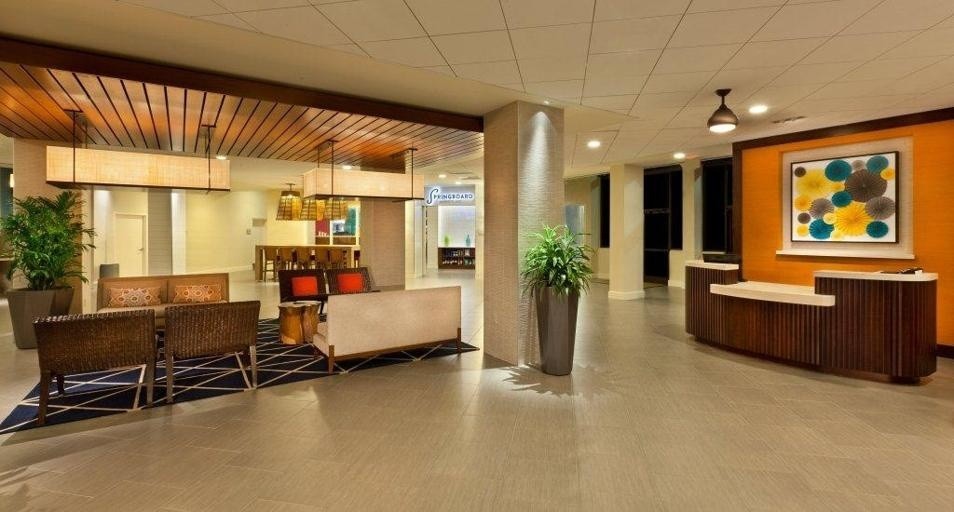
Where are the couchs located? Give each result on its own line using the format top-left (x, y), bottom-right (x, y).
top-left (97, 273), bottom-right (229, 329)
top-left (313, 287), bottom-right (461, 374)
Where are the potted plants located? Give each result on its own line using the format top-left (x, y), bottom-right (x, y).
top-left (0, 192), bottom-right (96, 348)
top-left (519, 222), bottom-right (598, 375)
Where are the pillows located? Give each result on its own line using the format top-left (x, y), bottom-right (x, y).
top-left (108, 287), bottom-right (161, 308)
top-left (172, 284), bottom-right (222, 303)
top-left (291, 276), bottom-right (318, 296)
top-left (337, 272), bottom-right (364, 292)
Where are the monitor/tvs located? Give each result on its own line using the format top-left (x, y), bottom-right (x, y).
top-left (703, 254), bottom-right (742, 281)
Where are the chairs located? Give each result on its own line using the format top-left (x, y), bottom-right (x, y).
top-left (166, 301), bottom-right (260, 403)
top-left (326, 268), bottom-right (380, 301)
top-left (32, 310), bottom-right (155, 424)
top-left (263, 247), bottom-right (343, 282)
top-left (279, 269), bottom-right (327, 316)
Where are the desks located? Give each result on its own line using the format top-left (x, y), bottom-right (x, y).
top-left (277, 300), bottom-right (320, 345)
top-left (256, 246), bottom-right (360, 280)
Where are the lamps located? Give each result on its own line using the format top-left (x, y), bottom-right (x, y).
top-left (278, 183), bottom-right (349, 220)
top-left (707, 90), bottom-right (738, 132)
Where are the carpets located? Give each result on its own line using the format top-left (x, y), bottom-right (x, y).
top-left (0, 320), bottom-right (480, 433)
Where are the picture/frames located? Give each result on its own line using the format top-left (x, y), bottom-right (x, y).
top-left (791, 152), bottom-right (899, 243)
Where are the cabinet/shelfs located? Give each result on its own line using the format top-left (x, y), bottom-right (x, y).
top-left (438, 248), bottom-right (475, 269)
